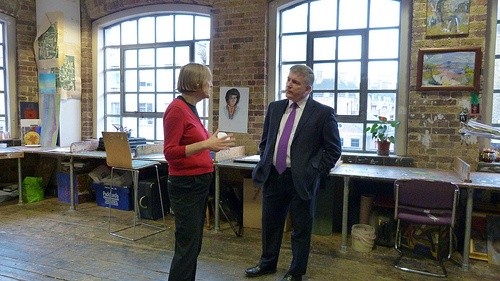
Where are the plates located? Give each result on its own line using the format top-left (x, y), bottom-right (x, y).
top-left (25, 145), bottom-right (41, 147)
top-left (24, 131), bottom-right (40, 144)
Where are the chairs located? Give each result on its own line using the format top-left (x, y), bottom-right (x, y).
top-left (394, 177), bottom-right (459, 279)
top-left (101, 131), bottom-right (167, 241)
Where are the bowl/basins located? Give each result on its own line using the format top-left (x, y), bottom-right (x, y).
top-left (0, 143), bottom-right (7, 149)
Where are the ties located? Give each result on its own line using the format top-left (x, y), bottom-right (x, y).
top-left (276, 102), bottom-right (298, 176)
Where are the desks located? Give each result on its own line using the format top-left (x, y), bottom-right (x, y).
top-left (0, 145), bottom-right (500, 273)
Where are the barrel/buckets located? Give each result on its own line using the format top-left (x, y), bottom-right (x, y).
top-left (351, 224), bottom-right (376, 252)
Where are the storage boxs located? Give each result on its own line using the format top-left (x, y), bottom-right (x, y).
top-left (57, 172), bottom-right (94, 204)
top-left (94, 183), bottom-right (132, 211)
top-left (242, 178), bottom-right (291, 232)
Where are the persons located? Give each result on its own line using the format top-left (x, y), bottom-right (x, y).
top-left (162, 62), bottom-right (236, 281)
top-left (246, 64), bottom-right (342, 281)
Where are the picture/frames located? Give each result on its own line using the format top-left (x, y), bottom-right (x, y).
top-left (416, 46), bottom-right (482, 92)
top-left (217, 86), bottom-right (250, 133)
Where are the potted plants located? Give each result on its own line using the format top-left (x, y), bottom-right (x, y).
top-left (365, 113), bottom-right (400, 155)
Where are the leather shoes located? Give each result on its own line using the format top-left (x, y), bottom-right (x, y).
top-left (281, 271), bottom-right (301, 281)
top-left (246, 266), bottom-right (277, 277)
